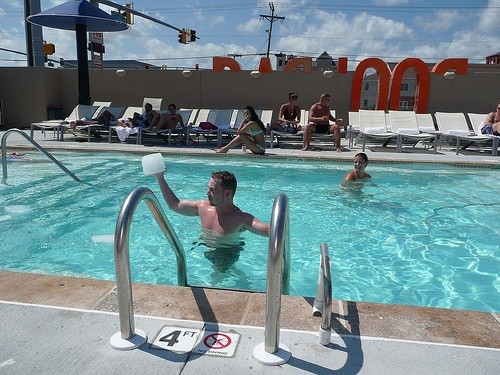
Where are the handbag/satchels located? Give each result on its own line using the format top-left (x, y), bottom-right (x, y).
top-left (200, 122), bottom-right (218, 130)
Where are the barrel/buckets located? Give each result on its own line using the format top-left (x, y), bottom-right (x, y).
top-left (142, 153), bottom-right (167, 176)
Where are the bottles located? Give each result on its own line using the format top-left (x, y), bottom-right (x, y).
top-left (242, 144), bottom-right (246, 152)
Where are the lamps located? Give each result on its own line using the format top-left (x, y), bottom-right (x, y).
top-left (250, 71), bottom-right (261, 79)
top-left (115, 70), bottom-right (127, 77)
top-left (443, 71), bottom-right (456, 81)
top-left (181, 70), bottom-right (192, 78)
top-left (322, 70), bottom-right (335, 79)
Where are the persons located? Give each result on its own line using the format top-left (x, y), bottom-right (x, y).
top-left (69, 102), bottom-right (197, 146)
top-left (300, 93), bottom-right (344, 153)
top-left (345, 153), bottom-right (372, 182)
top-left (271, 92), bottom-right (301, 131)
top-left (154, 167), bottom-right (270, 245)
top-left (481, 102), bottom-right (500, 137)
top-left (214, 106), bottom-right (266, 154)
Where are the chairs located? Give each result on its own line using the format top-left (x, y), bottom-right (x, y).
top-left (30, 98), bottom-right (500, 156)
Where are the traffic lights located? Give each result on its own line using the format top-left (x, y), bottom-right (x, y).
top-left (178, 28), bottom-right (186, 43)
top-left (122, 4), bottom-right (130, 25)
top-left (188, 29), bottom-right (197, 42)
top-left (145, 64), bottom-right (149, 69)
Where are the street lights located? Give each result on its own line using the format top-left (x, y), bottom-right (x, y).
top-left (265, 29), bottom-right (271, 56)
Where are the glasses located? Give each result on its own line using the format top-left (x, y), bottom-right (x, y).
top-left (497, 108), bottom-right (500, 110)
top-left (292, 99), bottom-right (299, 101)
